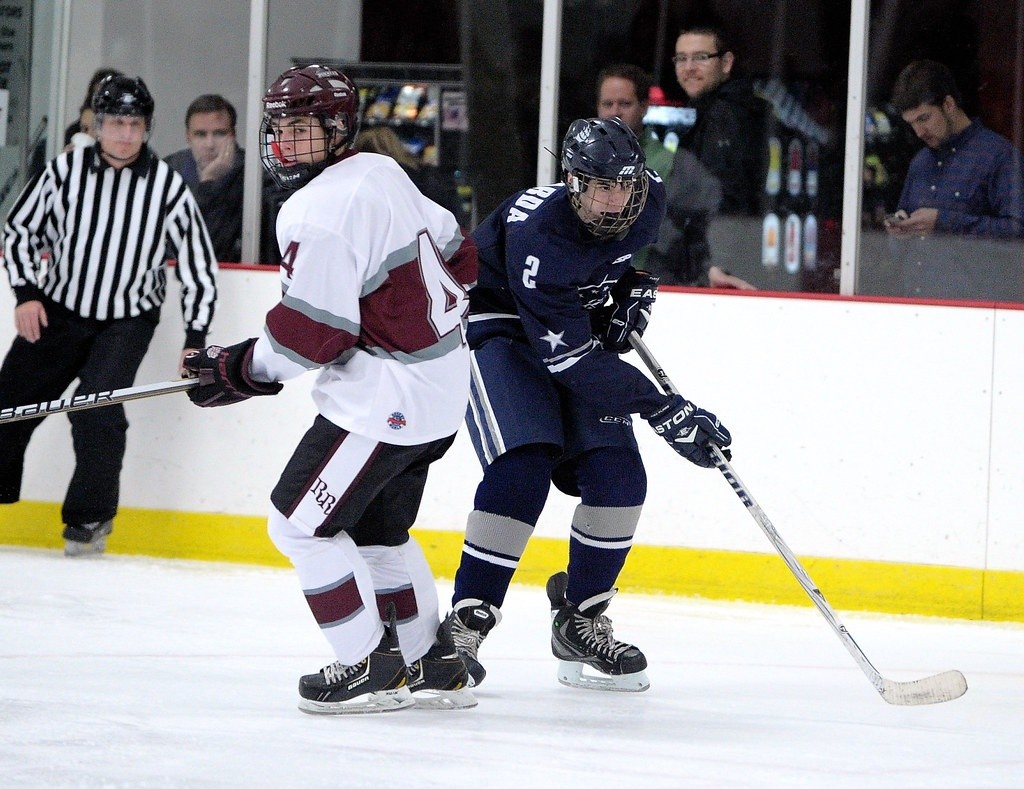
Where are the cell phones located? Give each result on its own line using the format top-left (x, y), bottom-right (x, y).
top-left (885, 213), bottom-right (904, 224)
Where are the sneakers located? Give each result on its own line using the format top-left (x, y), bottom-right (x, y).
top-left (405, 612), bottom-right (478, 710)
top-left (62, 518), bottom-right (113, 556)
top-left (447, 598), bottom-right (502, 689)
top-left (295, 601), bottom-right (416, 713)
top-left (546, 571), bottom-right (651, 692)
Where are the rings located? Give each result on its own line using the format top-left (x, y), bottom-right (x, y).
top-left (225, 152), bottom-right (230, 156)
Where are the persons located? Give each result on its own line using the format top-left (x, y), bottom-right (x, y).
top-left (163, 92), bottom-right (265, 260)
top-left (27, 68), bottom-right (171, 180)
top-left (182, 64), bottom-right (481, 714)
top-left (0, 74), bottom-right (220, 556)
top-left (593, 66), bottom-right (753, 289)
top-left (440, 117), bottom-right (732, 693)
top-left (354, 127), bottom-right (461, 223)
top-left (878, 55), bottom-right (1024, 239)
top-left (661, 24), bottom-right (771, 219)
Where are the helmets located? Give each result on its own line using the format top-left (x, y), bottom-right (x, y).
top-left (257, 63), bottom-right (360, 190)
top-left (559, 115), bottom-right (650, 241)
top-left (92, 75), bottom-right (154, 115)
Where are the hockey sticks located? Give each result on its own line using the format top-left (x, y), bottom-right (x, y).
top-left (0, 374), bottom-right (200, 426)
top-left (630, 329), bottom-right (969, 707)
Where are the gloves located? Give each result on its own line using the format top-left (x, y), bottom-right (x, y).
top-left (648, 394), bottom-right (732, 468)
top-left (181, 337), bottom-right (284, 408)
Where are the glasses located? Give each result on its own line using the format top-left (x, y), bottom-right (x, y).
top-left (672, 53), bottom-right (721, 63)
